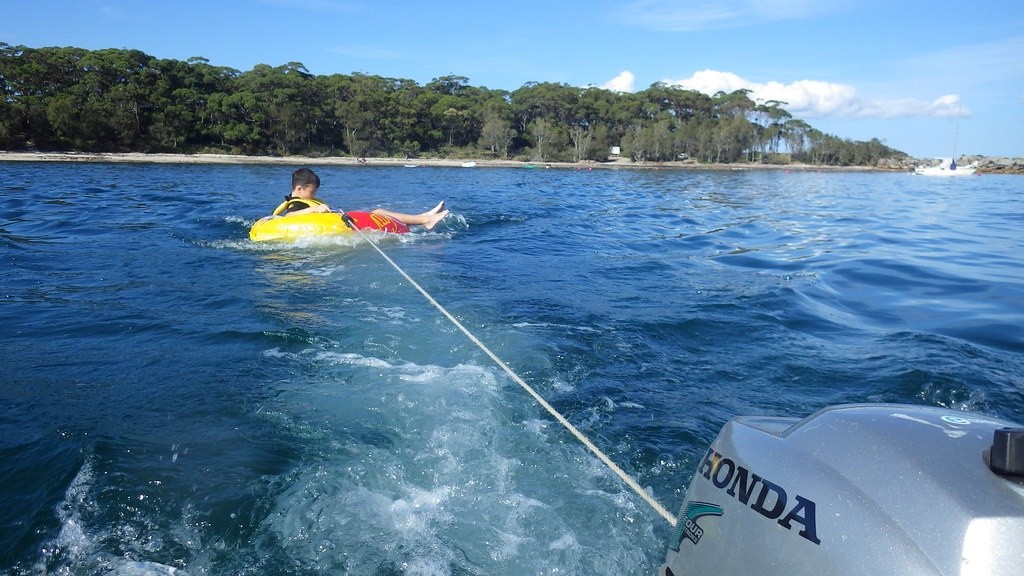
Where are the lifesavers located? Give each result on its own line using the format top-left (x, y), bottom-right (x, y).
top-left (243, 204), bottom-right (411, 253)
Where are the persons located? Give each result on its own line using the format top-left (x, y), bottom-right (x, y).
top-left (284, 168), bottom-right (449, 230)
